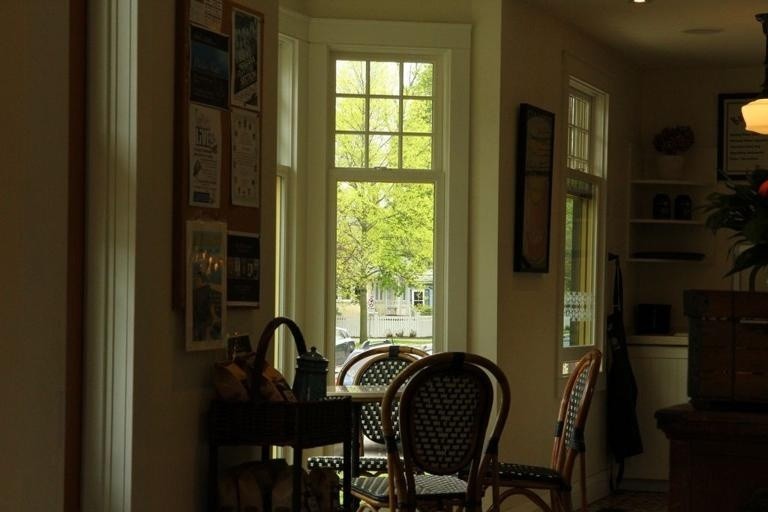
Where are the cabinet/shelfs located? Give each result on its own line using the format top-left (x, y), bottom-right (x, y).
top-left (682, 287), bottom-right (768, 408)
top-left (623, 143), bottom-right (707, 268)
top-left (206, 396), bottom-right (355, 510)
top-left (615, 344), bottom-right (691, 493)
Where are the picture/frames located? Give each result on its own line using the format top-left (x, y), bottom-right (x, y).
top-left (716, 91), bottom-right (768, 182)
top-left (511, 100), bottom-right (555, 274)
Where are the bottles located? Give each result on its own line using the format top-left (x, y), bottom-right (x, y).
top-left (652, 193), bottom-right (671, 219)
top-left (291, 347), bottom-right (329, 402)
top-left (674, 195), bottom-right (692, 220)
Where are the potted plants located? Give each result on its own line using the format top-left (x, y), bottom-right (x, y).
top-left (649, 124), bottom-right (695, 179)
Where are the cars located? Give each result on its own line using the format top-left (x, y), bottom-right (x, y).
top-left (335, 327), bottom-right (355, 367)
top-left (335, 341), bottom-right (432, 387)
